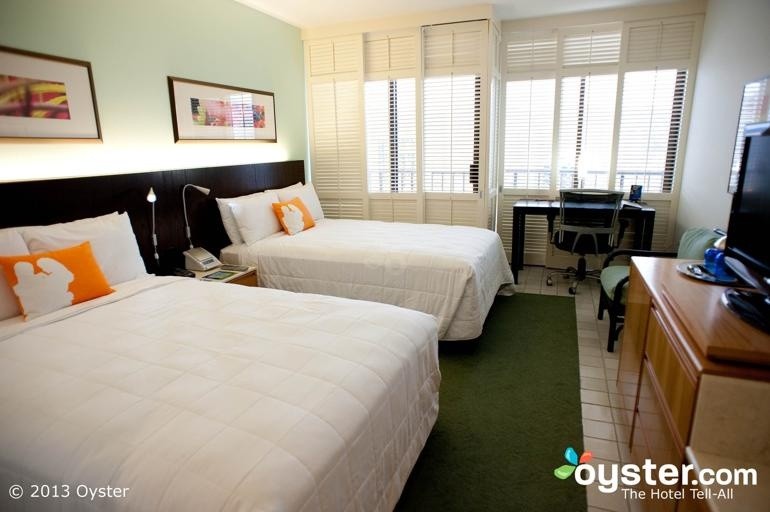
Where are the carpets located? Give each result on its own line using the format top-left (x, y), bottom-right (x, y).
top-left (391, 293), bottom-right (592, 510)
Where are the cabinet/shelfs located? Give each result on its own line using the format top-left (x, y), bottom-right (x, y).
top-left (615, 257), bottom-right (768, 511)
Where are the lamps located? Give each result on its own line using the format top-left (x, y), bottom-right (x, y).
top-left (146, 187), bottom-right (159, 265)
top-left (182, 184), bottom-right (209, 249)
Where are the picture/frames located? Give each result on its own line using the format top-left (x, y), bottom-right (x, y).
top-left (0, 42), bottom-right (103, 141)
top-left (167, 75), bottom-right (279, 144)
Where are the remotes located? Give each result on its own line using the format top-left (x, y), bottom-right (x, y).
top-left (221, 264), bottom-right (249, 272)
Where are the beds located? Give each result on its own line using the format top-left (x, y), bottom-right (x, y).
top-left (220, 217), bottom-right (516, 354)
top-left (1, 273), bottom-right (442, 512)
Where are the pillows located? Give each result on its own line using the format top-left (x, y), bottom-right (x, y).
top-left (216, 181), bottom-right (326, 246)
top-left (0, 211), bottom-right (148, 323)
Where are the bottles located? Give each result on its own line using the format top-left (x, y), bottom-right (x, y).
top-left (629, 185), bottom-right (642, 204)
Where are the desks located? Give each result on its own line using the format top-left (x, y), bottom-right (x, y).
top-left (511, 199), bottom-right (656, 285)
top-left (174, 263), bottom-right (258, 286)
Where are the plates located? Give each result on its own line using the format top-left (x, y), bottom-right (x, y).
top-left (675, 261), bottom-right (737, 284)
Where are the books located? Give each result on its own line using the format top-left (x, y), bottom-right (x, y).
top-left (220, 263), bottom-right (250, 274)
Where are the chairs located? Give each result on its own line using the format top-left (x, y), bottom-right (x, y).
top-left (544, 189), bottom-right (625, 295)
top-left (598, 226), bottom-right (727, 352)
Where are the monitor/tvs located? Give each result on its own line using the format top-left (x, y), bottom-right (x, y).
top-left (720, 119), bottom-right (770, 334)
